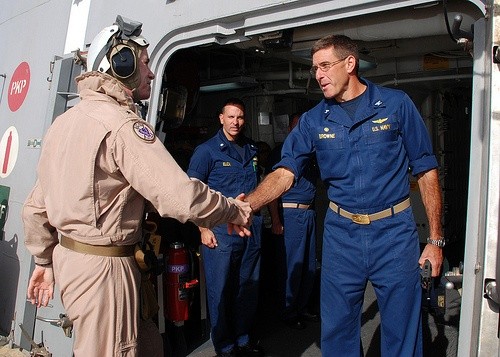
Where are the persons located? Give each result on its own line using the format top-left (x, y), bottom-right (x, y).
top-left (227, 34), bottom-right (446, 357)
top-left (267, 112), bottom-right (319, 328)
top-left (187, 101), bottom-right (267, 357)
top-left (22, 16), bottom-right (253, 357)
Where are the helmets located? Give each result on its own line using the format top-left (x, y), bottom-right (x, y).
top-left (86, 25), bottom-right (150, 73)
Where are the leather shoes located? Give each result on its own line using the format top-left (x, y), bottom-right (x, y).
top-left (297, 310), bottom-right (320, 322)
top-left (279, 316), bottom-right (305, 329)
top-left (216, 342), bottom-right (265, 357)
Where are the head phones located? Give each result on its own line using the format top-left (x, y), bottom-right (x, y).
top-left (110, 44), bottom-right (137, 79)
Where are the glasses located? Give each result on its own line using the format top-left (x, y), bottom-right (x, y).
top-left (113, 15), bottom-right (143, 40)
top-left (309, 57), bottom-right (347, 76)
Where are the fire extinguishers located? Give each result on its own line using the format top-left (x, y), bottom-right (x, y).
top-left (165, 240), bottom-right (193, 328)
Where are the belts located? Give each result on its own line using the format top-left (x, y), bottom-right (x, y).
top-left (280, 202), bottom-right (313, 210)
top-left (329, 197), bottom-right (413, 225)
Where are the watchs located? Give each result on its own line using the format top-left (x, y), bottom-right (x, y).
top-left (426, 236), bottom-right (445, 247)
